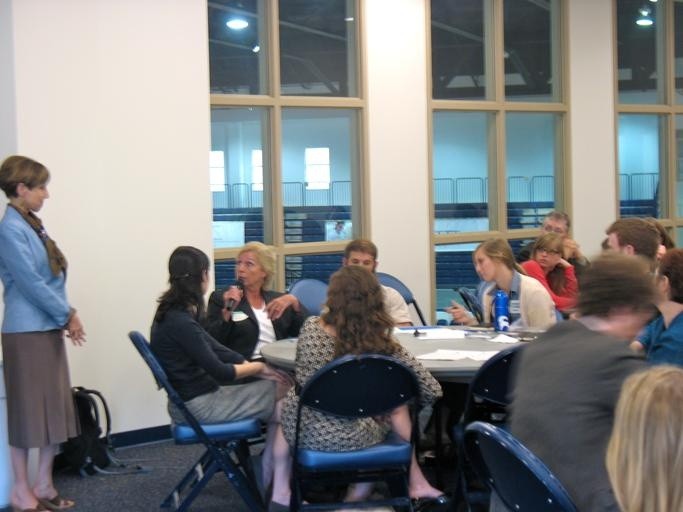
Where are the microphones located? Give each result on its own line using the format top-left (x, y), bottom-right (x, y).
top-left (227, 279), bottom-right (243, 311)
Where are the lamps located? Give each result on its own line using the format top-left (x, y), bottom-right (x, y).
top-left (634, 5), bottom-right (654, 27)
top-left (225, 3), bottom-right (248, 30)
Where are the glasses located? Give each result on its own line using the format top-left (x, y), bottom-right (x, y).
top-left (536, 245), bottom-right (559, 257)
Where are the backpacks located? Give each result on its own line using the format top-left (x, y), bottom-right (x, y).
top-left (60, 387), bottom-right (110, 476)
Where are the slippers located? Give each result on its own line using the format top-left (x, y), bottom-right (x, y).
top-left (411, 488), bottom-right (456, 510)
top-left (12, 501), bottom-right (47, 512)
top-left (39, 492), bottom-right (75, 510)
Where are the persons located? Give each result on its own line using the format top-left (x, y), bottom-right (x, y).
top-left (0, 155), bottom-right (86, 512)
top-left (600, 215), bottom-right (683, 367)
top-left (207, 242), bottom-right (311, 364)
top-left (343, 240), bottom-right (413, 328)
top-left (604, 364), bottom-right (683, 512)
top-left (327, 221), bottom-right (350, 241)
top-left (149, 247), bottom-right (293, 504)
top-left (518, 232), bottom-right (579, 309)
top-left (442, 238), bottom-right (556, 334)
top-left (518, 211), bottom-right (591, 278)
top-left (489, 252), bottom-right (661, 512)
top-left (280, 263), bottom-right (453, 511)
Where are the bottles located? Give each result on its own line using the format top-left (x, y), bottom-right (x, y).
top-left (494, 290), bottom-right (509, 332)
